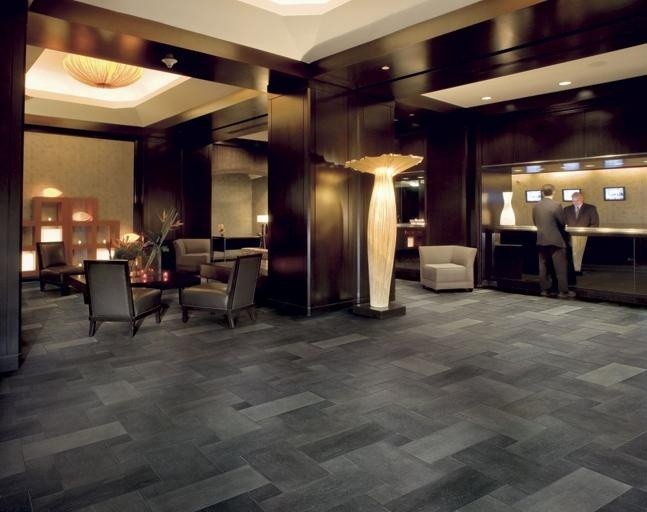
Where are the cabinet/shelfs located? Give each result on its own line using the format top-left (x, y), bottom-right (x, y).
top-left (19, 195), bottom-right (120, 281)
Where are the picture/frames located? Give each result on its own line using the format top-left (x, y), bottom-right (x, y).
top-left (602, 186), bottom-right (625, 201)
top-left (561, 188), bottom-right (580, 203)
top-left (524, 190), bottom-right (543, 202)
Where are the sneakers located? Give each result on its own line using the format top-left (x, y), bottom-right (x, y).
top-left (542, 290), bottom-right (558, 296)
top-left (558, 291), bottom-right (576, 297)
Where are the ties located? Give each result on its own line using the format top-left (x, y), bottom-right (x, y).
top-left (575, 206), bottom-right (580, 219)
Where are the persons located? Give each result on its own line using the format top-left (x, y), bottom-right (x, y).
top-left (530, 183), bottom-right (576, 299)
top-left (562, 192), bottom-right (599, 227)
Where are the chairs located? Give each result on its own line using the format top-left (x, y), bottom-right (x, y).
top-left (81, 259), bottom-right (163, 337)
top-left (417, 244), bottom-right (478, 293)
top-left (36, 240), bottom-right (84, 294)
top-left (179, 254), bottom-right (263, 331)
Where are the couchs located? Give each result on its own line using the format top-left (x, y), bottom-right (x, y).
top-left (174, 237), bottom-right (244, 278)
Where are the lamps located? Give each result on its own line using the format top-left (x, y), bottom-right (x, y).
top-left (343, 156), bottom-right (425, 319)
top-left (161, 52), bottom-right (180, 69)
top-left (499, 191), bottom-right (516, 226)
top-left (256, 214), bottom-right (268, 249)
top-left (60, 53), bottom-right (147, 91)
